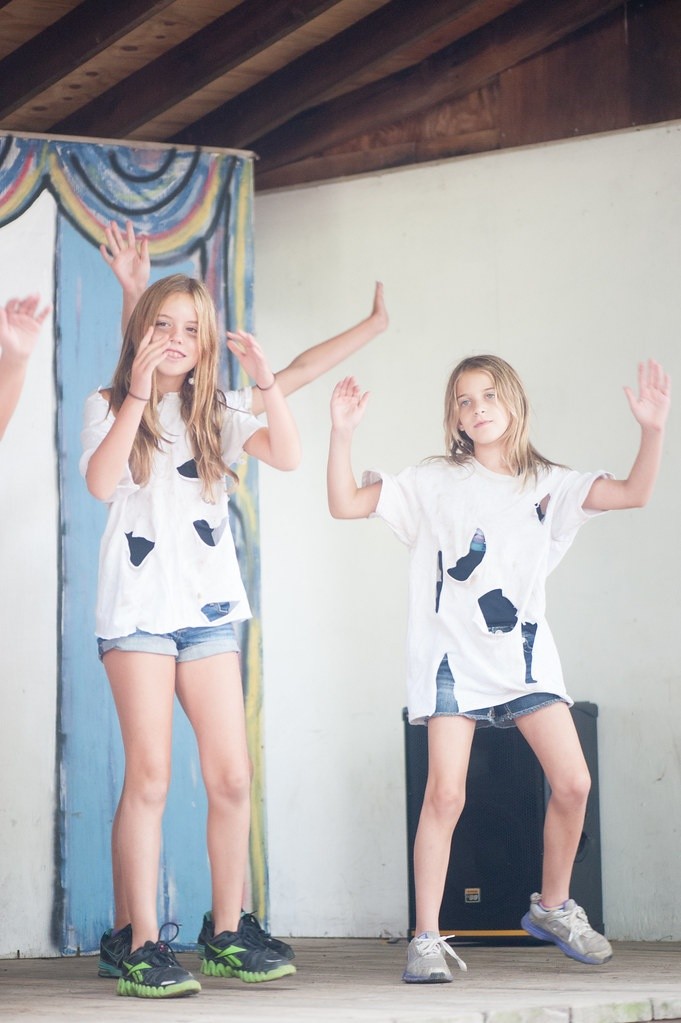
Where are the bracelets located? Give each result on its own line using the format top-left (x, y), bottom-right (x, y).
top-left (257, 373), bottom-right (276, 391)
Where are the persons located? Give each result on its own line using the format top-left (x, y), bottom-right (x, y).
top-left (77, 274), bottom-right (303, 998)
top-left (98, 221), bottom-right (390, 978)
top-left (327, 356), bottom-right (672, 984)
top-left (0, 293), bottom-right (51, 443)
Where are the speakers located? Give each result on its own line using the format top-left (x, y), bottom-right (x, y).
top-left (401, 702), bottom-right (605, 945)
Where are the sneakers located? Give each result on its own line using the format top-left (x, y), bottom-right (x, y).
top-left (201, 920), bottom-right (297, 982)
top-left (118, 920), bottom-right (202, 999)
top-left (402, 930), bottom-right (469, 983)
top-left (198, 907), bottom-right (296, 963)
top-left (522, 891), bottom-right (615, 964)
top-left (97, 922), bottom-right (136, 977)
